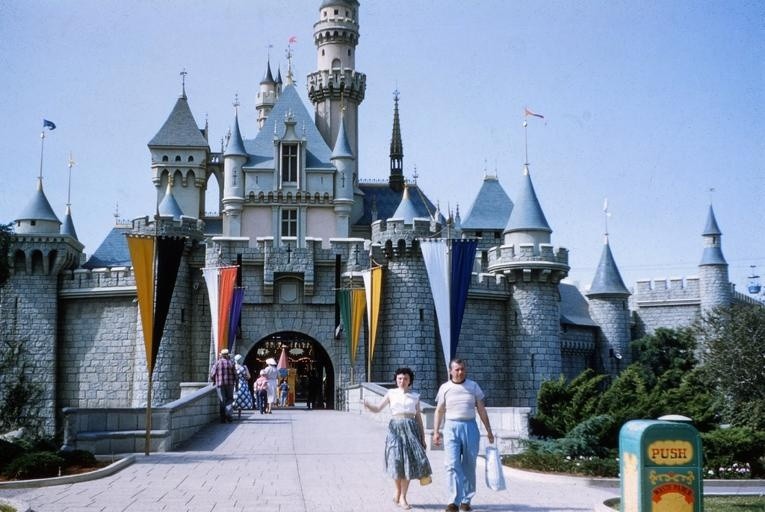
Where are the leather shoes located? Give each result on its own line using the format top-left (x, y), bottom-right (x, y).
top-left (459, 503), bottom-right (471, 512)
top-left (445, 503), bottom-right (458, 511)
top-left (392, 498), bottom-right (398, 508)
top-left (397, 502), bottom-right (410, 511)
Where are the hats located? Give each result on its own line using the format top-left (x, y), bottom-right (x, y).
top-left (265, 358), bottom-right (277, 365)
top-left (218, 348), bottom-right (232, 357)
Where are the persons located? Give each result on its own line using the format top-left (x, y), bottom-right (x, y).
top-left (211, 348), bottom-right (291, 423)
top-left (360, 366), bottom-right (434, 511)
top-left (433, 358), bottom-right (496, 511)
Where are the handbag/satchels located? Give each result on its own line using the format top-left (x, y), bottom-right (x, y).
top-left (483, 440), bottom-right (506, 492)
top-left (416, 445), bottom-right (433, 486)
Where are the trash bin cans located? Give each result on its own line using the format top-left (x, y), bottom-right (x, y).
top-left (619, 420), bottom-right (703, 512)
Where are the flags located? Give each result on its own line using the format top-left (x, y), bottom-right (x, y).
top-left (42, 119), bottom-right (57, 131)
top-left (523, 108), bottom-right (548, 124)
